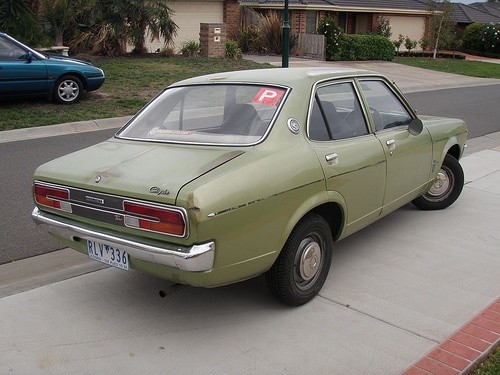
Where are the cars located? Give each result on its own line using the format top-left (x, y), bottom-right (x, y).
top-left (0, 31), bottom-right (106, 106)
top-left (32, 66), bottom-right (469, 307)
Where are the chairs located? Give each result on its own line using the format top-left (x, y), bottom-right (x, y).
top-left (218, 103), bottom-right (268, 136)
top-left (308, 101), bottom-right (356, 141)
